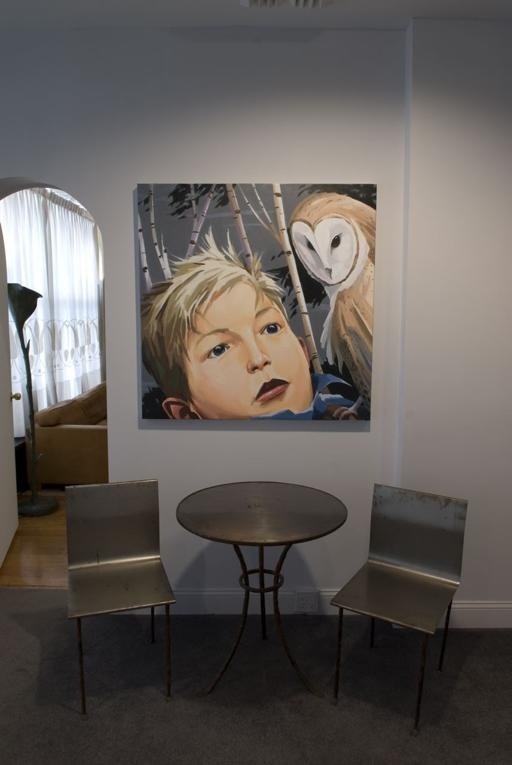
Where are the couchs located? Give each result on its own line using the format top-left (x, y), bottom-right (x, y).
top-left (25, 382), bottom-right (109, 488)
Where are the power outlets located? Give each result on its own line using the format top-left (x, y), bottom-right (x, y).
top-left (294, 589), bottom-right (320, 613)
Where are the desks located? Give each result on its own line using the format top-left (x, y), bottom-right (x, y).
top-left (176, 482), bottom-right (348, 699)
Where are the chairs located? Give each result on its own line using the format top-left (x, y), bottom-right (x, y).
top-left (63, 481), bottom-right (176, 714)
top-left (330, 482), bottom-right (469, 730)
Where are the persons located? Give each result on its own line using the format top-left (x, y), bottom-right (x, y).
top-left (142, 224), bottom-right (361, 422)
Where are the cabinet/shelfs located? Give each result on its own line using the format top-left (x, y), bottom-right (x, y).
top-left (14, 437), bottom-right (26, 492)
